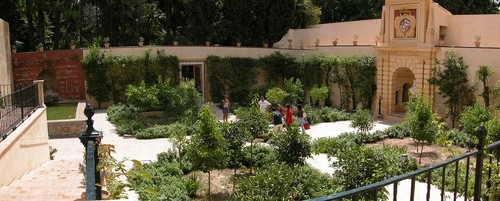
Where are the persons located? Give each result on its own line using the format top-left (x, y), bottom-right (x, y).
top-left (222, 95), bottom-right (229, 122)
top-left (277, 104), bottom-right (308, 134)
top-left (258, 96), bottom-right (272, 112)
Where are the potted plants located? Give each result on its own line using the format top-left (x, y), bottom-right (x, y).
top-left (475, 36), bottom-right (481, 46)
top-left (12, 45), bottom-right (16, 54)
top-left (37, 43), bottom-right (44, 51)
top-left (70, 40), bottom-right (76, 50)
top-left (104, 37), bottom-right (110, 47)
top-left (139, 37), bottom-right (144, 46)
